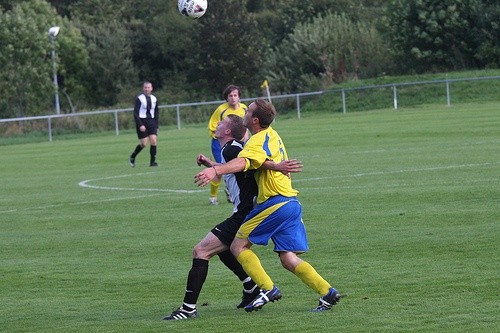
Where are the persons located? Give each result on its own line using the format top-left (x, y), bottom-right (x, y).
top-left (206, 85), bottom-right (250, 205)
top-left (160, 114), bottom-right (304, 320)
top-left (129, 80), bottom-right (160, 168)
top-left (193, 100), bottom-right (342, 312)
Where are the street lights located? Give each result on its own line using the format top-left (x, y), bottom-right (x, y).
top-left (49, 26), bottom-right (60, 114)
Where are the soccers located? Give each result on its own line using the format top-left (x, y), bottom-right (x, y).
top-left (177, 0), bottom-right (208, 18)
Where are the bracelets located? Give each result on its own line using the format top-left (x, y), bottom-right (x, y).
top-left (213, 166), bottom-right (217, 176)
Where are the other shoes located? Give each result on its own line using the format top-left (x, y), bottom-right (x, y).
top-left (210, 197), bottom-right (220, 205)
top-left (129, 155), bottom-right (136, 167)
top-left (224, 188), bottom-right (233, 203)
top-left (150, 163), bottom-right (159, 167)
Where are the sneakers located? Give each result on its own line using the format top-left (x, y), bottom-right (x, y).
top-left (313, 288), bottom-right (341, 311)
top-left (237, 284), bottom-right (261, 309)
top-left (163, 309), bottom-right (200, 320)
top-left (245, 285), bottom-right (282, 312)
